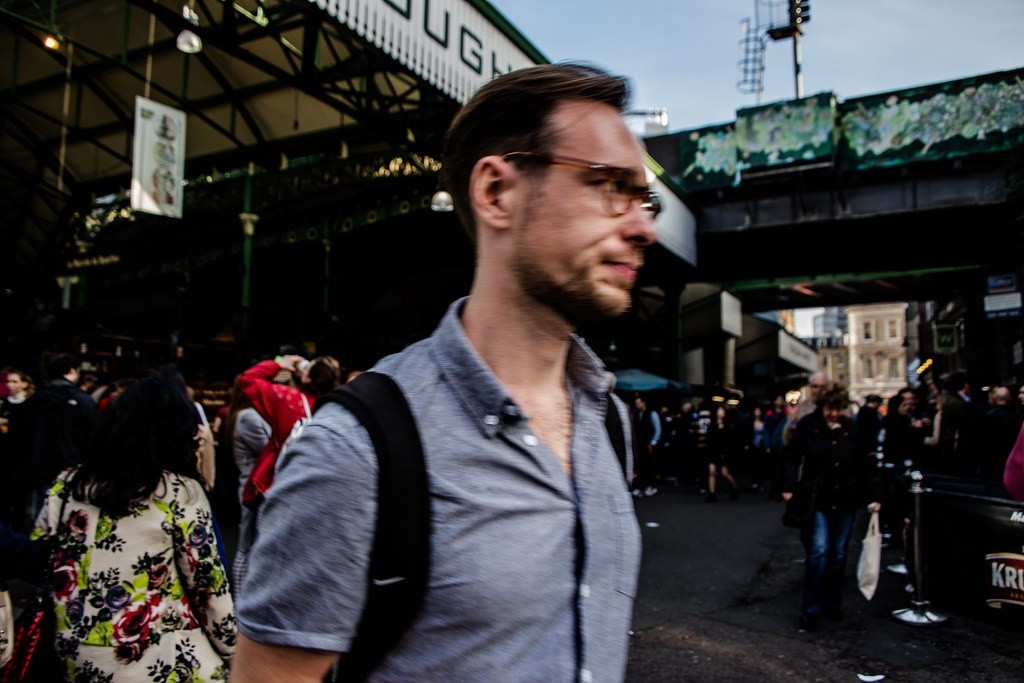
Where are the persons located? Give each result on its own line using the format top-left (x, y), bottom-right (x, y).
top-left (631, 368), bottom-right (1024, 631)
top-left (231, 63), bottom-right (664, 683)
top-left (0, 346), bottom-right (339, 683)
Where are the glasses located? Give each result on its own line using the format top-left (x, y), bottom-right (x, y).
top-left (500, 151), bottom-right (662, 220)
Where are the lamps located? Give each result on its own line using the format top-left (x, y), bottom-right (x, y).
top-left (175, 0), bottom-right (203, 53)
top-left (430, 176), bottom-right (455, 210)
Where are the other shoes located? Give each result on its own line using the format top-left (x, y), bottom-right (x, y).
top-left (643, 485), bottom-right (659, 497)
top-left (731, 487), bottom-right (741, 501)
top-left (631, 488), bottom-right (643, 498)
top-left (799, 610), bottom-right (820, 630)
top-left (705, 493), bottom-right (717, 503)
top-left (823, 602), bottom-right (844, 623)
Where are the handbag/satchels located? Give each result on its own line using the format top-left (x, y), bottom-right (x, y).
top-left (0, 586), bottom-right (57, 683)
top-left (856, 510), bottom-right (883, 602)
